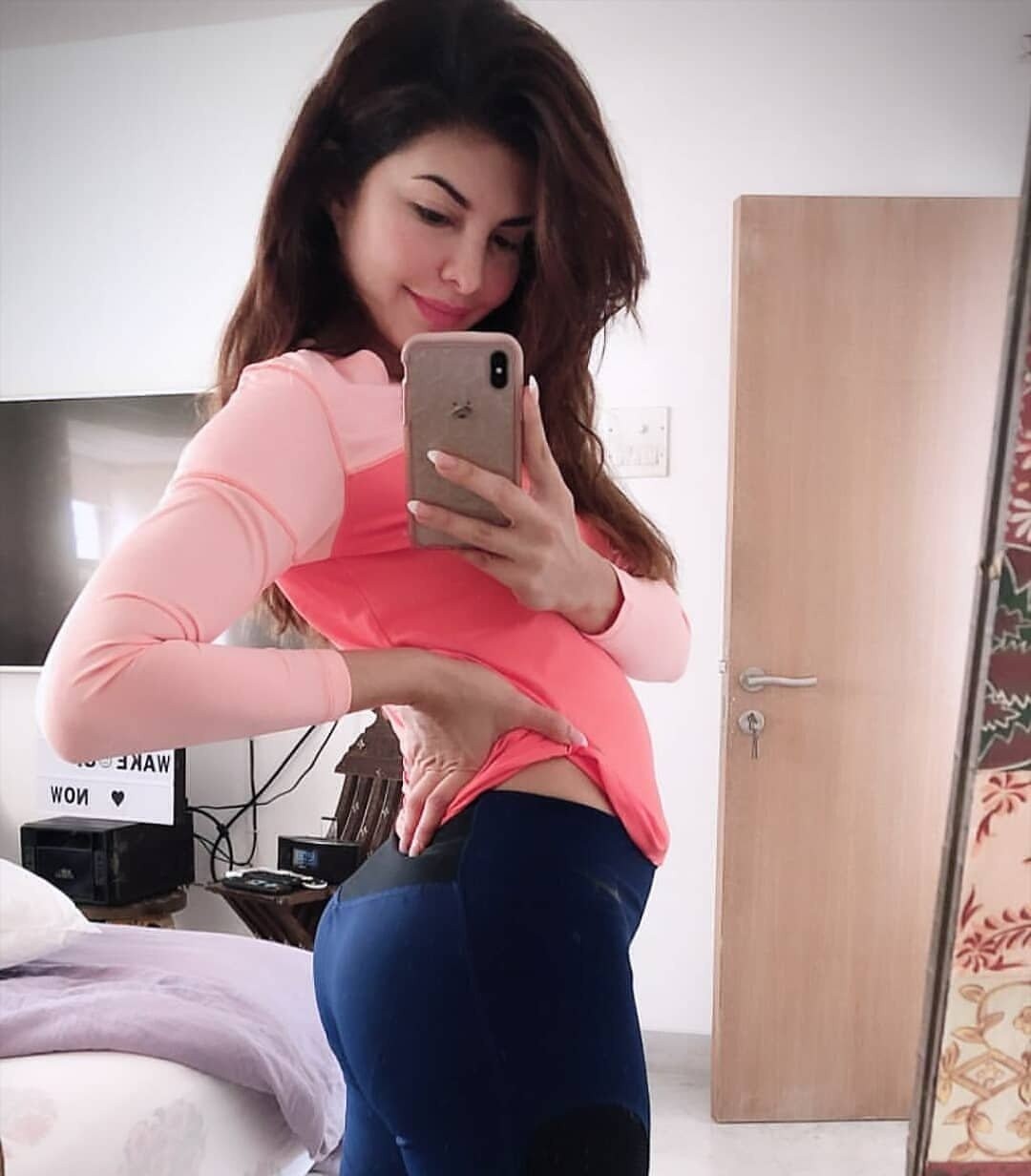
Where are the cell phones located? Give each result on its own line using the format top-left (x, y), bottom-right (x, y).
top-left (399, 332), bottom-right (525, 548)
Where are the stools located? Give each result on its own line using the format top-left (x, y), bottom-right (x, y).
top-left (73, 889), bottom-right (187, 930)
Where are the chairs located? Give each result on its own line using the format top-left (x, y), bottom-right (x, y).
top-left (204, 709), bottom-right (406, 950)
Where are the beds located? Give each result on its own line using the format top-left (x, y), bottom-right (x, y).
top-left (0, 857), bottom-right (346, 1176)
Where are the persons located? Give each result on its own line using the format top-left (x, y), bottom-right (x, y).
top-left (35, 0), bottom-right (692, 1176)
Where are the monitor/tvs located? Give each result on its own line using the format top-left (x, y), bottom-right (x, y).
top-left (0, 393), bottom-right (213, 673)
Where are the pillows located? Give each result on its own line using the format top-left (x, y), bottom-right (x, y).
top-left (0, 855), bottom-right (102, 979)
